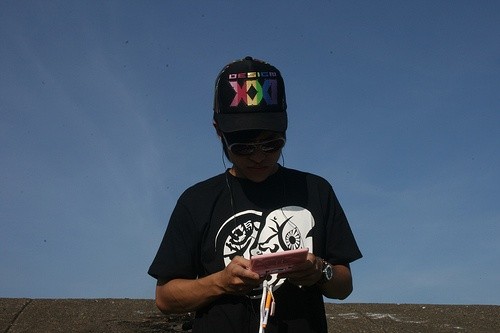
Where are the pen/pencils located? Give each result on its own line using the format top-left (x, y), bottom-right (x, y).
top-left (263, 285), bottom-right (273, 328)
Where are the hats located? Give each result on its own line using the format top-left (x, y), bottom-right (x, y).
top-left (213, 57), bottom-right (287, 131)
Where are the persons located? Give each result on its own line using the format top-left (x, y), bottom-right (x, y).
top-left (148, 56), bottom-right (363, 333)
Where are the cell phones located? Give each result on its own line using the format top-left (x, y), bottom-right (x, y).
top-left (251, 249), bottom-right (310, 272)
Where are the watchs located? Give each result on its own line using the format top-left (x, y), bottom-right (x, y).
top-left (307, 257), bottom-right (333, 292)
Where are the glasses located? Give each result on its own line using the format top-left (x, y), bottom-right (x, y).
top-left (221, 132), bottom-right (285, 154)
